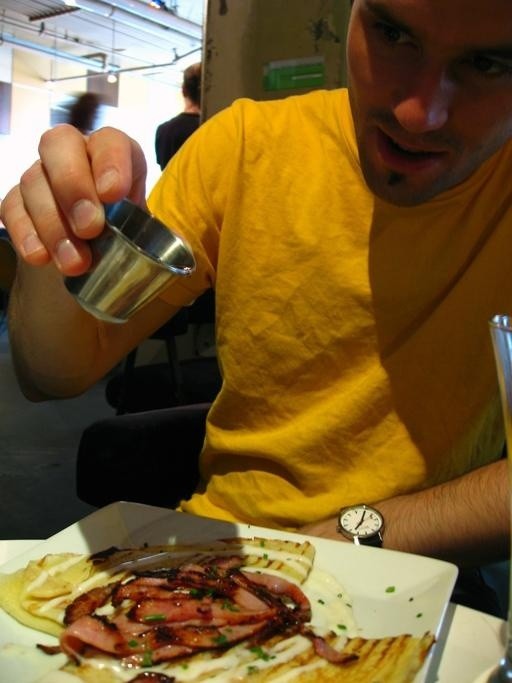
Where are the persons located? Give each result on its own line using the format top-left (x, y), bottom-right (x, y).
top-left (155, 62), bottom-right (201, 171)
top-left (71, 92), bottom-right (99, 136)
top-left (0, 0), bottom-right (512, 620)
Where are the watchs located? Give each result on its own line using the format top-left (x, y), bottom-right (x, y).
top-left (338, 504), bottom-right (385, 547)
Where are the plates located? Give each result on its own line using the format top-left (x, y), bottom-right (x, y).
top-left (1, 498), bottom-right (464, 683)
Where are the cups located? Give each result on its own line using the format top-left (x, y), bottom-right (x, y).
top-left (60, 190), bottom-right (196, 327)
